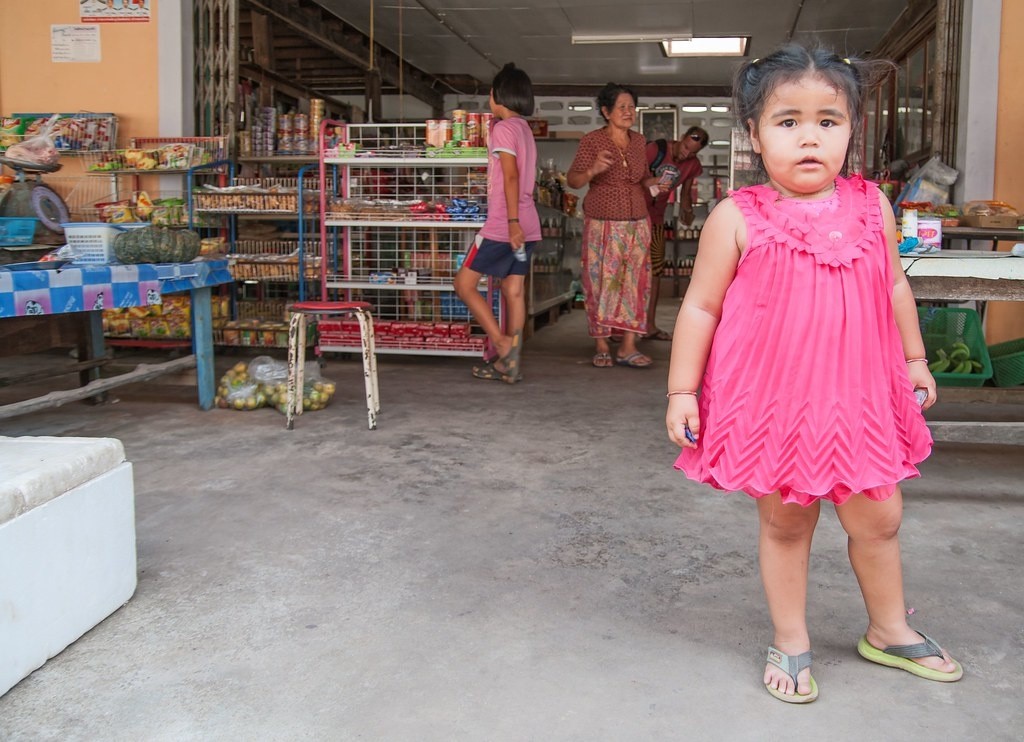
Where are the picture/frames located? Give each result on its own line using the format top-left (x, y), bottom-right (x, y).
top-left (639, 108), bottom-right (678, 141)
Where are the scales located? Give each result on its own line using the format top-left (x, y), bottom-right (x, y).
top-left (0, 156), bottom-right (72, 245)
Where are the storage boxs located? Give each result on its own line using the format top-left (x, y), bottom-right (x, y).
top-left (917, 307), bottom-right (993, 386)
top-left (59, 222), bottom-right (151, 264)
top-left (988, 338), bottom-right (1024, 388)
top-left (0, 216), bottom-right (41, 245)
top-left (961, 214), bottom-right (1018, 228)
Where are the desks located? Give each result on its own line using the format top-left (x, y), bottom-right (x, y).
top-left (0, 254), bottom-right (231, 422)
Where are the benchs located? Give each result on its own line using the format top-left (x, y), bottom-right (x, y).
top-left (0, 435), bottom-right (138, 698)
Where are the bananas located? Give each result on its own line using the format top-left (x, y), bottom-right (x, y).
top-left (929, 341), bottom-right (984, 373)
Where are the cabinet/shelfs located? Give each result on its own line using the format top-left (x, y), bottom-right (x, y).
top-left (658, 236), bottom-right (700, 278)
top-left (61, 116), bottom-right (578, 361)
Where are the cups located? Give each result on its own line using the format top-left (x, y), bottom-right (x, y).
top-left (902, 208), bottom-right (918, 241)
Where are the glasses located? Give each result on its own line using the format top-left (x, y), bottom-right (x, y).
top-left (690, 132), bottom-right (707, 146)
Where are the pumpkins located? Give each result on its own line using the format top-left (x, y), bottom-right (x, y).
top-left (112, 226), bottom-right (202, 263)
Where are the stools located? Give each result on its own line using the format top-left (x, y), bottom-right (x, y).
top-left (285, 301), bottom-right (380, 430)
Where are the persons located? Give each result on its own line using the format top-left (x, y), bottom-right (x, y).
top-left (608, 127), bottom-right (708, 342)
top-left (664, 36), bottom-right (964, 705)
top-left (346, 134), bottom-right (428, 201)
top-left (452, 62), bottom-right (542, 383)
top-left (565, 81), bottom-right (674, 368)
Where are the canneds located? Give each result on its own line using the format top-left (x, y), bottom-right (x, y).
top-left (426, 108), bottom-right (494, 147)
top-left (220, 318), bottom-right (316, 347)
top-left (239, 97), bottom-right (325, 155)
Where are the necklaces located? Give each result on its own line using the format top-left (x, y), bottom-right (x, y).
top-left (613, 138), bottom-right (628, 166)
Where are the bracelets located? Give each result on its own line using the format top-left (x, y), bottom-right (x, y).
top-left (667, 390), bottom-right (701, 397)
top-left (906, 359), bottom-right (929, 365)
top-left (507, 218), bottom-right (519, 223)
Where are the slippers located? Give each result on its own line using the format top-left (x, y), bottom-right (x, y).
top-left (641, 329), bottom-right (672, 341)
top-left (608, 335), bottom-right (623, 342)
top-left (499, 329), bottom-right (523, 385)
top-left (616, 352), bottom-right (654, 368)
top-left (765, 646), bottom-right (818, 703)
top-left (858, 629), bottom-right (962, 683)
top-left (472, 360), bottom-right (522, 381)
top-left (591, 352), bottom-right (614, 368)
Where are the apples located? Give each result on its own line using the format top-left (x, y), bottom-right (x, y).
top-left (213, 362), bottom-right (335, 412)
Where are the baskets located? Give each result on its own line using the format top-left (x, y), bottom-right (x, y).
top-left (988, 338), bottom-right (1024, 387)
top-left (0, 217), bottom-right (40, 246)
top-left (60, 223), bottom-right (151, 264)
top-left (916, 307), bottom-right (993, 388)
